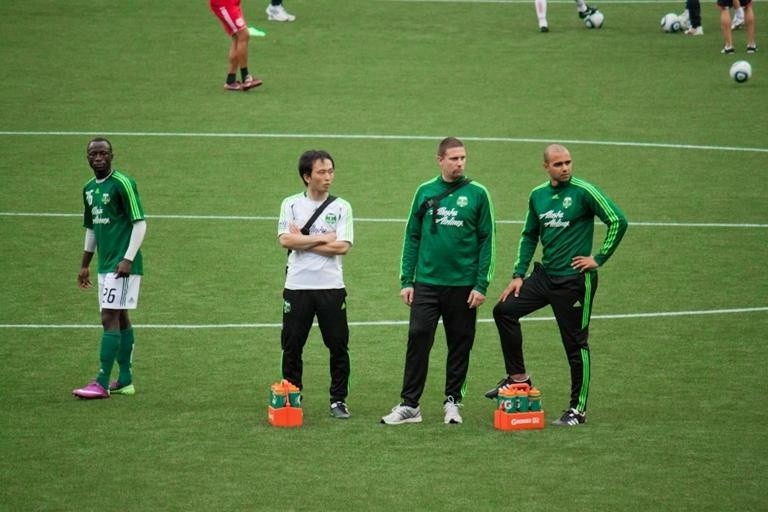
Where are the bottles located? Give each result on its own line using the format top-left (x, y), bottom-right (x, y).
top-left (269, 380), bottom-right (301, 408)
top-left (497, 384), bottom-right (543, 414)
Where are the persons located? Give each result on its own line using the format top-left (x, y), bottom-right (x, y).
top-left (535, 1), bottom-right (598, 33)
top-left (265, 1), bottom-right (294, 22)
top-left (683, 0), bottom-right (746, 36)
top-left (209, 1), bottom-right (262, 92)
top-left (484, 145), bottom-right (628, 426)
top-left (277, 151), bottom-right (354, 419)
top-left (382, 137), bottom-right (496, 433)
top-left (717, 0), bottom-right (758, 54)
top-left (73, 139), bottom-right (147, 400)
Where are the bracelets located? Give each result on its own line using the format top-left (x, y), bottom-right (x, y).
top-left (513, 274), bottom-right (523, 278)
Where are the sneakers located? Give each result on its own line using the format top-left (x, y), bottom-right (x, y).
top-left (684, 26), bottom-right (703, 35)
top-left (224, 76), bottom-right (262, 89)
top-left (552, 408), bottom-right (585, 426)
top-left (265, 3), bottom-right (296, 22)
top-left (71, 382), bottom-right (135, 399)
top-left (329, 401), bottom-right (350, 418)
top-left (731, 11), bottom-right (748, 28)
top-left (381, 405), bottom-right (422, 424)
top-left (443, 395), bottom-right (463, 424)
top-left (579, 6), bottom-right (596, 18)
top-left (719, 46), bottom-right (757, 54)
top-left (485, 376), bottom-right (531, 397)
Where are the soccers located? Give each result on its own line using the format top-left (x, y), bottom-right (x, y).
top-left (659, 13), bottom-right (681, 32)
top-left (585, 12), bottom-right (604, 28)
top-left (729, 62), bottom-right (752, 83)
